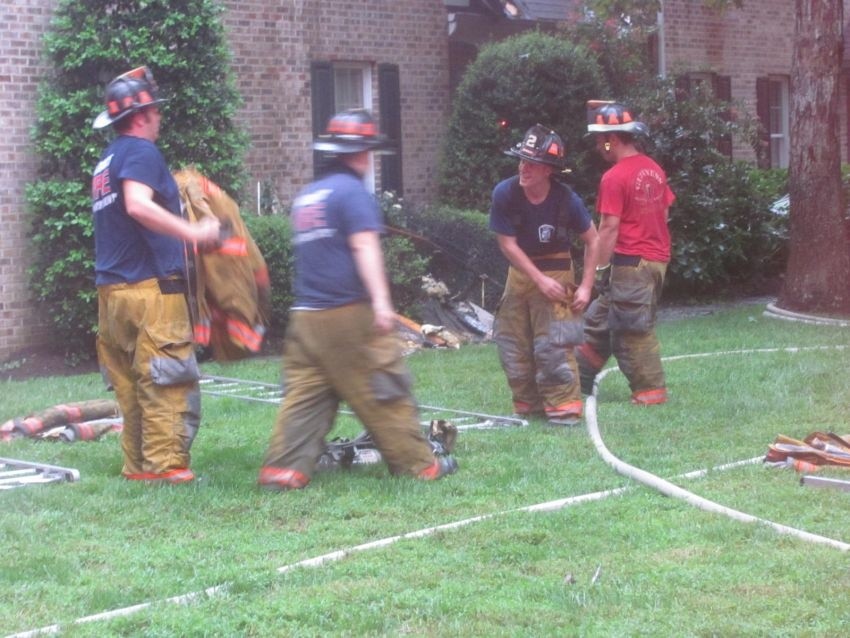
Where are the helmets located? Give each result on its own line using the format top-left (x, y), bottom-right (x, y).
top-left (503, 124), bottom-right (566, 175)
top-left (582, 101), bottom-right (651, 137)
top-left (92, 77), bottom-right (168, 130)
top-left (314, 108), bottom-right (399, 155)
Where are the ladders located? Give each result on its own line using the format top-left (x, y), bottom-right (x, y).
top-left (199, 373), bottom-right (528, 432)
top-left (0, 457), bottom-right (80, 490)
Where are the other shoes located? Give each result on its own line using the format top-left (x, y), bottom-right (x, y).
top-left (435, 456), bottom-right (458, 480)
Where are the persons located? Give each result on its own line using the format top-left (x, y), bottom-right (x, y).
top-left (92, 66), bottom-right (219, 485)
top-left (255, 106), bottom-right (456, 490)
top-left (579, 106), bottom-right (674, 406)
top-left (491, 123), bottom-right (601, 424)
